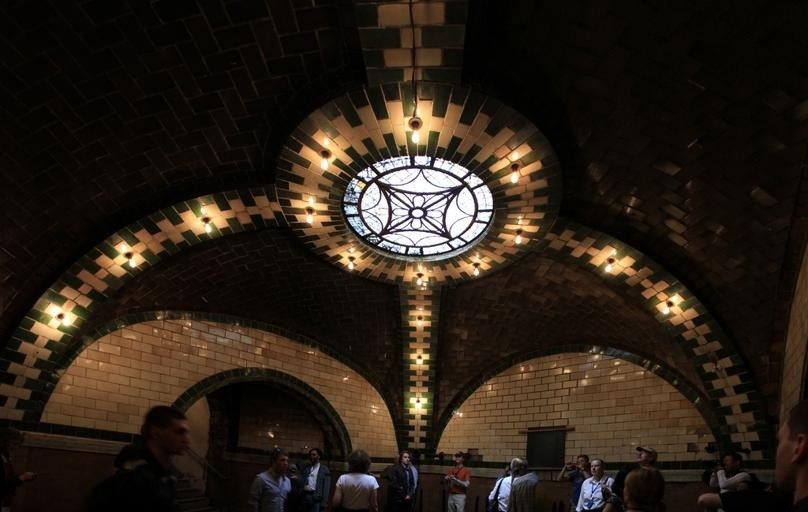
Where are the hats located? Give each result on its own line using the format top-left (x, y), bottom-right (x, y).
top-left (454, 451), bottom-right (464, 457)
top-left (635, 445), bottom-right (658, 458)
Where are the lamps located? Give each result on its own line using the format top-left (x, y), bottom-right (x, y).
top-left (123, 252), bottom-right (137, 269)
top-left (302, 206), bottom-right (314, 226)
top-left (509, 163), bottom-right (520, 184)
top-left (344, 255), bottom-right (358, 271)
top-left (55, 313), bottom-right (70, 327)
top-left (405, 102), bottom-right (424, 144)
top-left (513, 229), bottom-right (524, 245)
top-left (199, 216), bottom-right (212, 232)
top-left (604, 259), bottom-right (615, 273)
top-left (412, 313), bottom-right (426, 410)
top-left (416, 273), bottom-right (423, 286)
top-left (471, 262), bottom-right (480, 277)
top-left (318, 147), bottom-right (332, 174)
top-left (660, 300), bottom-right (675, 317)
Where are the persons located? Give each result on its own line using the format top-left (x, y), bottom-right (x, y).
top-left (774, 404), bottom-right (808, 512)
top-left (697, 452), bottom-right (765, 511)
top-left (0, 426), bottom-right (34, 512)
top-left (83, 405), bottom-right (191, 512)
top-left (488, 457), bottom-right (540, 512)
top-left (111, 433), bottom-right (141, 474)
top-left (444, 451), bottom-right (471, 512)
top-left (249, 445), bottom-right (419, 512)
top-left (557, 447), bottom-right (665, 512)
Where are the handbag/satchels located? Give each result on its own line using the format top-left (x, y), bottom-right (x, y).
top-left (488, 478), bottom-right (503, 511)
